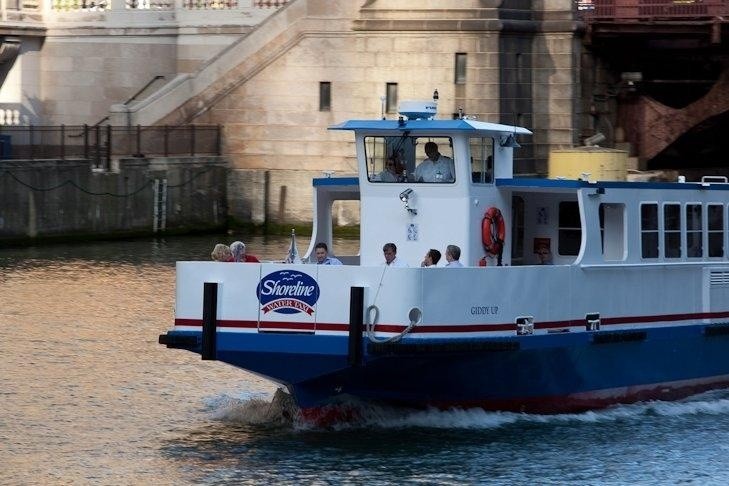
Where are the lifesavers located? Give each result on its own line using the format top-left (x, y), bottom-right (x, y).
top-left (482, 207), bottom-right (506, 254)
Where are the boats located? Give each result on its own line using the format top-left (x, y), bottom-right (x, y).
top-left (158, 89), bottom-right (729, 427)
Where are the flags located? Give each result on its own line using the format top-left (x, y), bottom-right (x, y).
top-left (284, 232), bottom-right (303, 264)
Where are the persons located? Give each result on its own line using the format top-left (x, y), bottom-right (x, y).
top-left (222, 240), bottom-right (262, 262)
top-left (370, 158), bottom-right (403, 182)
top-left (413, 142), bottom-right (456, 183)
top-left (211, 244), bottom-right (231, 262)
top-left (443, 245), bottom-right (465, 267)
top-left (380, 242), bottom-right (409, 267)
top-left (312, 243), bottom-right (342, 265)
top-left (419, 248), bottom-right (441, 267)
